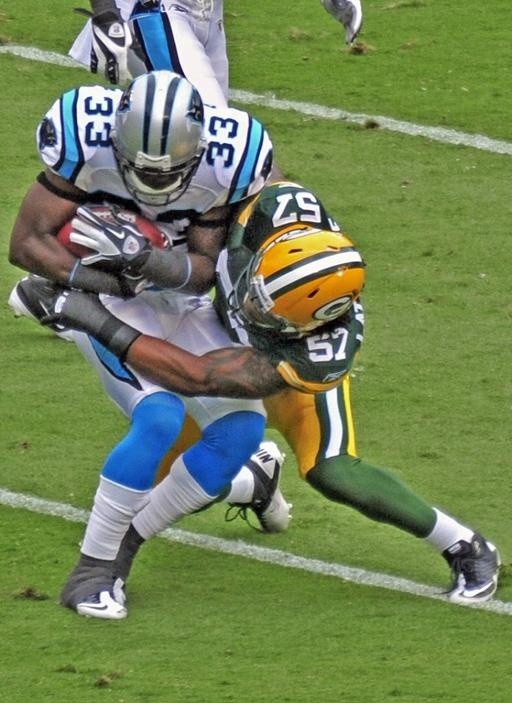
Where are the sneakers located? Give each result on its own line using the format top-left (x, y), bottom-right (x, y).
top-left (441, 534), bottom-right (501, 602)
top-left (8, 276), bottom-right (74, 342)
top-left (62, 553), bottom-right (133, 620)
top-left (227, 441), bottom-right (293, 532)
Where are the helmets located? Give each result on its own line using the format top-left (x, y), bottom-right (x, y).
top-left (110, 70), bottom-right (204, 171)
top-left (255, 224), bottom-right (366, 334)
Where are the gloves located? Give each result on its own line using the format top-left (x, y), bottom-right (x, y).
top-left (321, 0), bottom-right (362, 44)
top-left (29, 275), bottom-right (142, 359)
top-left (71, 203), bottom-right (190, 297)
top-left (90, 11), bottom-right (147, 84)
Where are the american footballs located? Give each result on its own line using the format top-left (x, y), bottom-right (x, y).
top-left (53, 204), bottom-right (171, 276)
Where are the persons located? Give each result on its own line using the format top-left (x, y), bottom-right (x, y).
top-left (7, 68), bottom-right (279, 624)
top-left (67, 0), bottom-right (361, 112)
top-left (15, 168), bottom-right (501, 608)
